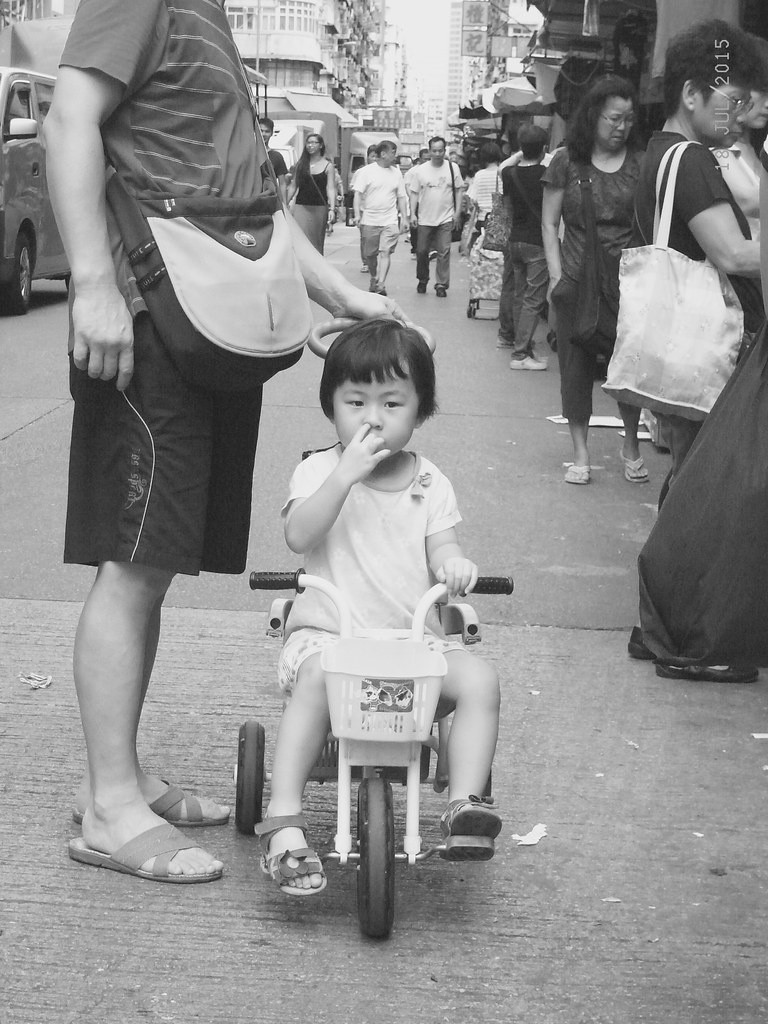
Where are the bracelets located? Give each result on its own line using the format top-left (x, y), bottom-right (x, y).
top-left (328, 208), bottom-right (337, 215)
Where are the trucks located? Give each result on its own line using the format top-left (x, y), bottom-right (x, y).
top-left (337, 125), bottom-right (427, 208)
top-left (0, 13), bottom-right (73, 319)
top-left (258, 110), bottom-right (340, 175)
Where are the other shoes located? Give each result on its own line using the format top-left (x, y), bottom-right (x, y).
top-left (361, 264), bottom-right (369, 272)
top-left (428, 250), bottom-right (437, 261)
top-left (436, 288), bottom-right (446, 297)
top-left (411, 252), bottom-right (416, 260)
top-left (417, 283), bottom-right (426, 293)
top-left (626, 627), bottom-right (759, 682)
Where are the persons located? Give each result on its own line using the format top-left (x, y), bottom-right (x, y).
top-left (41, 0), bottom-right (408, 884)
top-left (539, 76), bottom-right (649, 484)
top-left (497, 122), bottom-right (551, 370)
top-left (259, 118), bottom-right (287, 204)
top-left (287, 134), bottom-right (336, 255)
top-left (466, 141), bottom-right (503, 320)
top-left (255, 319), bottom-right (503, 895)
top-left (351, 137), bottom-right (467, 298)
top-left (708, 33), bottom-right (768, 333)
top-left (630, 17), bottom-right (768, 682)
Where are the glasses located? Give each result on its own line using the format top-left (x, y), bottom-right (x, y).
top-left (699, 78), bottom-right (754, 114)
top-left (306, 141), bottom-right (320, 146)
top-left (601, 113), bottom-right (635, 127)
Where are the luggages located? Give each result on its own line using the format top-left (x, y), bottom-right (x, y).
top-left (468, 226), bottom-right (504, 316)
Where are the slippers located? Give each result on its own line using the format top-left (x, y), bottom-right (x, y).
top-left (74, 779), bottom-right (231, 827)
top-left (510, 356), bottom-right (548, 370)
top-left (69, 824), bottom-right (223, 884)
top-left (620, 449), bottom-right (649, 483)
top-left (565, 465), bottom-right (591, 484)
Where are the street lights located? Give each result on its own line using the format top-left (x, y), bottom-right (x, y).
top-left (336, 41), bottom-right (358, 104)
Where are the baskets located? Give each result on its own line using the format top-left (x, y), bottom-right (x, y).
top-left (320, 639), bottom-right (448, 742)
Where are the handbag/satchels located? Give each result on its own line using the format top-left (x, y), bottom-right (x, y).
top-left (483, 192), bottom-right (503, 250)
top-left (602, 244), bottom-right (745, 421)
top-left (576, 233), bottom-right (619, 355)
top-left (116, 192), bottom-right (311, 389)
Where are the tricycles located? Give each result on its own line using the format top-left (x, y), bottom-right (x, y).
top-left (225, 568), bottom-right (518, 944)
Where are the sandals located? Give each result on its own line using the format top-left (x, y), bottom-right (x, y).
top-left (255, 813), bottom-right (327, 896)
top-left (441, 795), bottom-right (502, 861)
